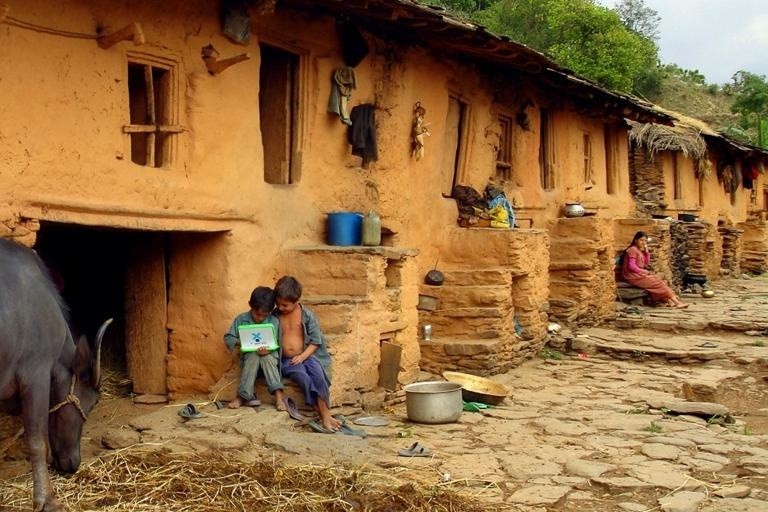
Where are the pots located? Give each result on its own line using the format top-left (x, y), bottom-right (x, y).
top-left (402, 381), bottom-right (464, 423)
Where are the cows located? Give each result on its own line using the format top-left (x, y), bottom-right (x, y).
top-left (0, 238), bottom-right (115, 512)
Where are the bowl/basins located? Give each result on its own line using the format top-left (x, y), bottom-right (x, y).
top-left (443, 370), bottom-right (513, 405)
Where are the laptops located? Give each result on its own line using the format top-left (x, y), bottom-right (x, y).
top-left (236, 322), bottom-right (280, 354)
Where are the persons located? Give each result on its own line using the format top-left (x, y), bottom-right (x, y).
top-left (271, 275), bottom-right (342, 433)
top-left (618, 229), bottom-right (690, 310)
top-left (221, 284), bottom-right (288, 413)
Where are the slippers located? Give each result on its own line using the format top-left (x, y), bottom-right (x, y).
top-left (398, 441), bottom-right (429, 456)
top-left (310, 420), bottom-right (366, 438)
top-left (178, 403), bottom-right (208, 418)
top-left (243, 392), bottom-right (261, 406)
top-left (272, 395), bottom-right (305, 421)
